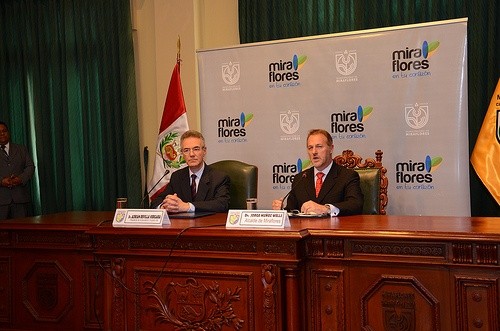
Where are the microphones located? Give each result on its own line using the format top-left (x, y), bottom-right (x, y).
top-left (281, 172), bottom-right (308, 216)
top-left (140, 170), bottom-right (169, 208)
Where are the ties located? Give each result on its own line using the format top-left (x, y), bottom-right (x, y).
top-left (1, 145), bottom-right (9, 161)
top-left (191, 174), bottom-right (197, 201)
top-left (316, 172), bottom-right (325, 198)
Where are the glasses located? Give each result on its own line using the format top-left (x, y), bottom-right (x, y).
top-left (182, 147), bottom-right (203, 154)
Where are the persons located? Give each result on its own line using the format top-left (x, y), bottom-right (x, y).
top-left (149, 129), bottom-right (231, 213)
top-left (272, 129), bottom-right (364, 216)
top-left (0, 121), bottom-right (35, 219)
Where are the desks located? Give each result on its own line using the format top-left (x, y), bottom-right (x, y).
top-left (0, 210), bottom-right (500, 331)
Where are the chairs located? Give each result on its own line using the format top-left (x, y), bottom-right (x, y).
top-left (207, 160), bottom-right (258, 209)
top-left (332, 148), bottom-right (389, 215)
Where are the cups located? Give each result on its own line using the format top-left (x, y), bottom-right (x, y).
top-left (116, 198), bottom-right (127, 209)
top-left (246, 199), bottom-right (257, 210)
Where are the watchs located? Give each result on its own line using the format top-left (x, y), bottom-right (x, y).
top-left (325, 206), bottom-right (331, 216)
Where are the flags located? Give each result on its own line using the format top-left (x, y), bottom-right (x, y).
top-left (149, 61), bottom-right (189, 205)
